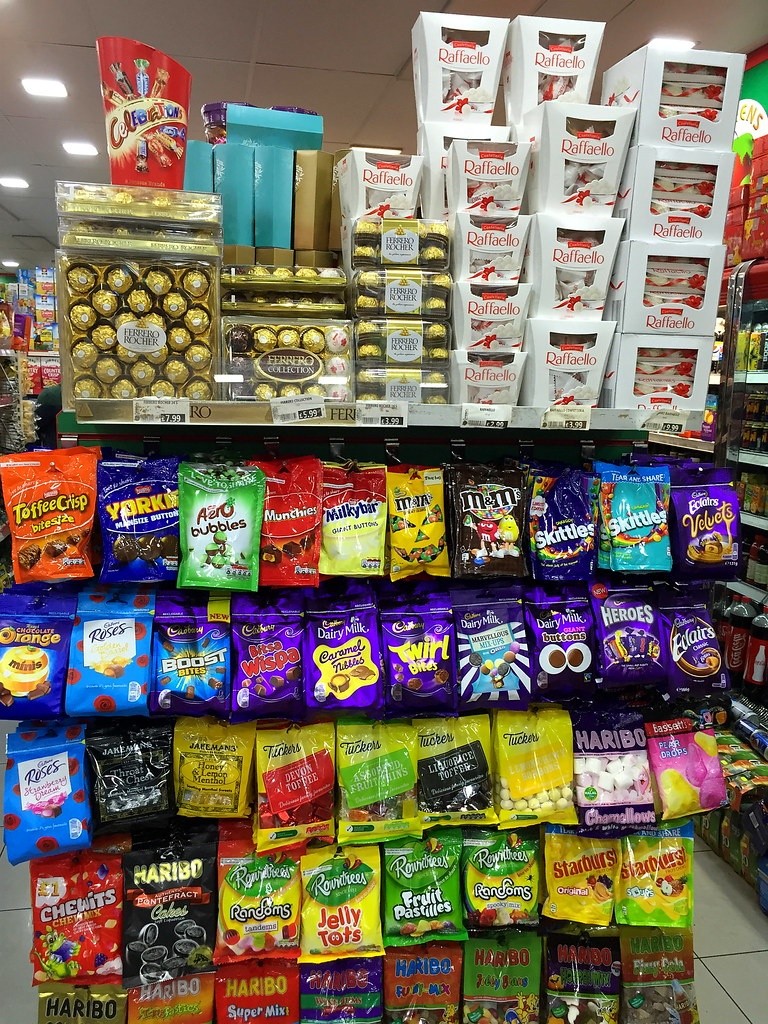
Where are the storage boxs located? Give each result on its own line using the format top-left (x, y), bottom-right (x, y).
top-left (8, 11), bottom-right (747, 412)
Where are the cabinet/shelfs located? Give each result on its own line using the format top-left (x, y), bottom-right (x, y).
top-left (0, 348), bottom-right (59, 544)
top-left (648, 258), bottom-right (768, 733)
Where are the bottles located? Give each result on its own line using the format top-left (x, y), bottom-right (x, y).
top-left (753, 542), bottom-right (768, 589)
top-left (713, 590), bottom-right (731, 629)
top-left (745, 534), bottom-right (764, 585)
top-left (740, 537), bottom-right (751, 581)
top-left (715, 594), bottom-right (742, 658)
top-left (741, 606), bottom-right (768, 705)
top-left (752, 646), bottom-right (766, 683)
top-left (723, 599), bottom-right (756, 689)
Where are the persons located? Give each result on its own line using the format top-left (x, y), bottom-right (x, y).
top-left (35, 384), bottom-right (62, 449)
